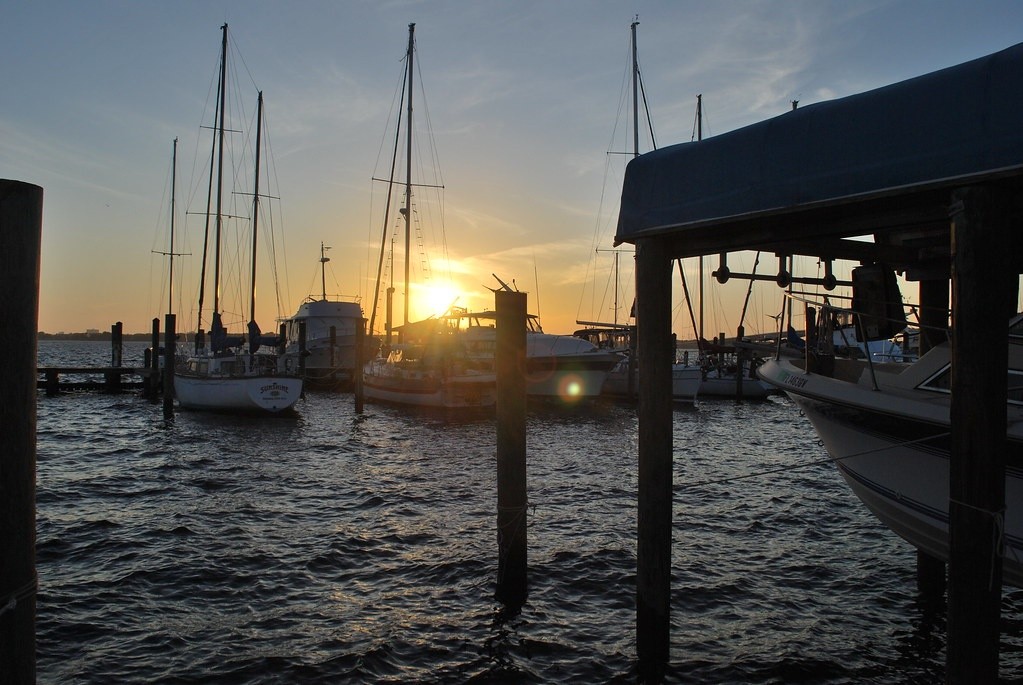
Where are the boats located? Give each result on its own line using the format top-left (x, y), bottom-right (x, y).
top-left (146, 17), bottom-right (308, 416)
top-left (755, 286), bottom-right (1023, 592)
top-left (277, 241), bottom-right (383, 387)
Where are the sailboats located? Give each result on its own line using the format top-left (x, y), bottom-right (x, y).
top-left (353, 9), bottom-right (904, 405)
